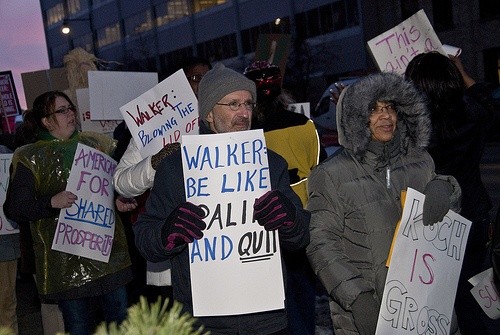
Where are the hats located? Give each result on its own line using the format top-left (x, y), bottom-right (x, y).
top-left (198, 64), bottom-right (257, 127)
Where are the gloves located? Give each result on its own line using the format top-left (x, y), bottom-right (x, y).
top-left (151, 142), bottom-right (181, 171)
top-left (350, 292), bottom-right (380, 335)
top-left (423, 180), bottom-right (453, 226)
top-left (254, 188), bottom-right (297, 231)
top-left (161, 201), bottom-right (206, 250)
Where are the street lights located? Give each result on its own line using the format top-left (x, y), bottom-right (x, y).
top-left (62, 12), bottom-right (100, 71)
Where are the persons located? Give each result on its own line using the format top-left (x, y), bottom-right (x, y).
top-left (0, 49), bottom-right (500, 335)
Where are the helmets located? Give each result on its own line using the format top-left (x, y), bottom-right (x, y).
top-left (254, 79), bottom-right (282, 97)
top-left (242, 60), bottom-right (282, 78)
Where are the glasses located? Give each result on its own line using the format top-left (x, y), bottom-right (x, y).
top-left (45, 106), bottom-right (76, 117)
top-left (187, 74), bottom-right (203, 82)
top-left (371, 104), bottom-right (395, 113)
top-left (216, 100), bottom-right (256, 110)
